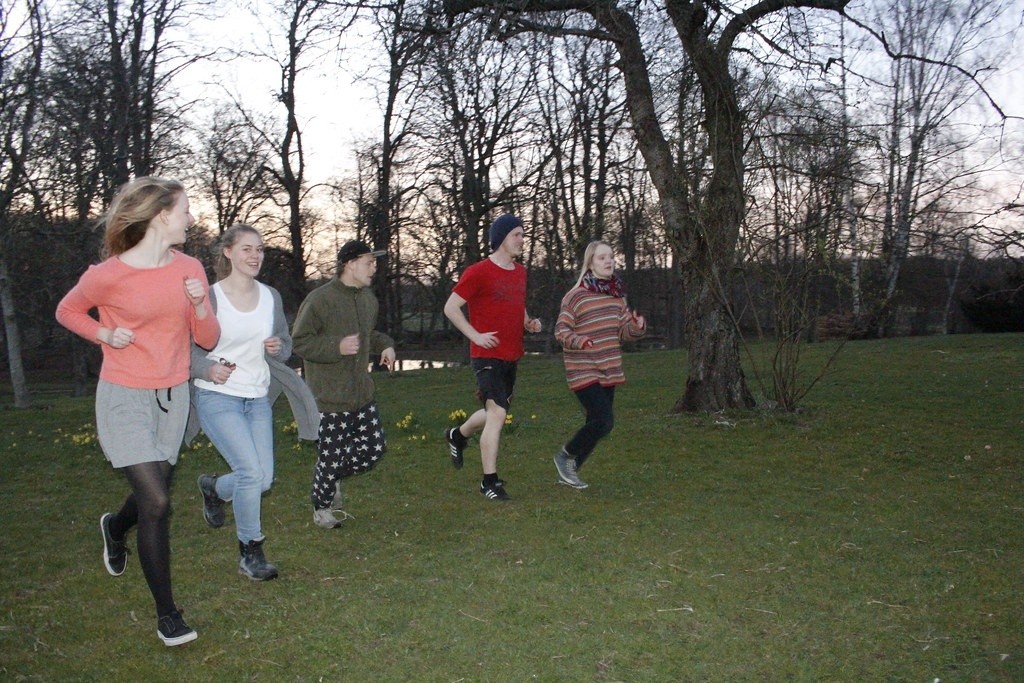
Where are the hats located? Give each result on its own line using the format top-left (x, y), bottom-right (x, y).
top-left (338, 240), bottom-right (388, 265)
top-left (489, 214), bottom-right (525, 251)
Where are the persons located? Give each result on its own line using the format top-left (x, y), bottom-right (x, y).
top-left (295, 239), bottom-right (397, 531)
top-left (443, 214), bottom-right (542, 503)
top-left (191, 221), bottom-right (319, 581)
top-left (550, 238), bottom-right (649, 491)
top-left (55, 174), bottom-right (221, 647)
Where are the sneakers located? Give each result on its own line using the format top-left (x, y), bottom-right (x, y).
top-left (553, 447), bottom-right (589, 489)
top-left (330, 480), bottom-right (343, 510)
top-left (479, 479), bottom-right (511, 502)
top-left (237, 539), bottom-right (280, 582)
top-left (155, 610), bottom-right (199, 648)
top-left (99, 511), bottom-right (128, 578)
top-left (312, 508), bottom-right (343, 530)
top-left (446, 428), bottom-right (467, 470)
top-left (196, 473), bottom-right (228, 528)
